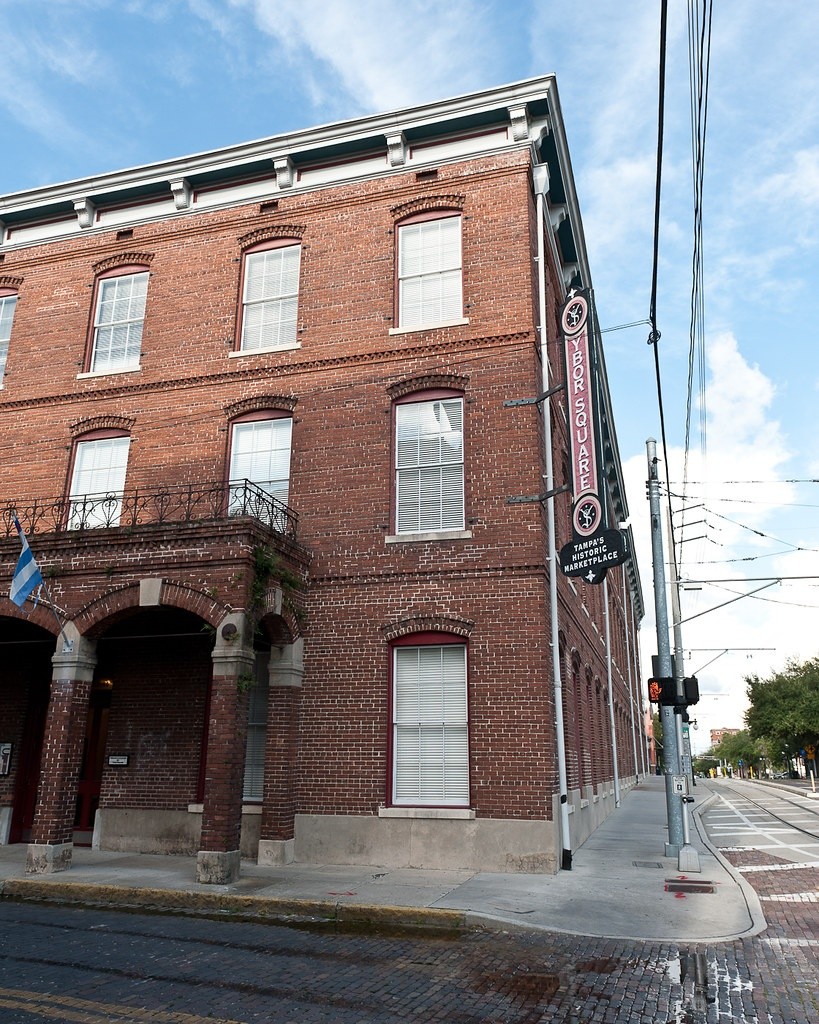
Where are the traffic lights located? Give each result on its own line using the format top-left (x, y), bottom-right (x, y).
top-left (648, 678), bottom-right (677, 703)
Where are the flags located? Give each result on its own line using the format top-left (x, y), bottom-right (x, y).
top-left (8, 515), bottom-right (42, 608)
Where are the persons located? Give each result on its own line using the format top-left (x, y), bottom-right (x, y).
top-left (697, 770), bottom-right (700, 776)
top-left (709, 767), bottom-right (717, 778)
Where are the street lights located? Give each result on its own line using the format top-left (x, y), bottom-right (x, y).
top-left (687, 718), bottom-right (699, 787)
